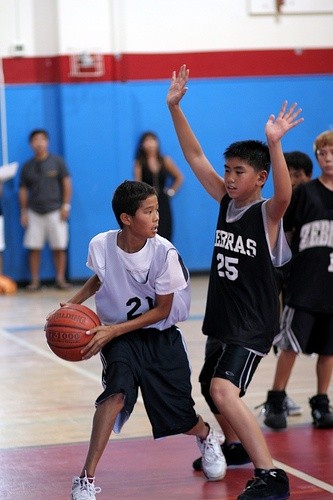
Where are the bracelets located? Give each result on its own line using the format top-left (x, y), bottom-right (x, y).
top-left (20, 205), bottom-right (27, 210)
top-left (61, 202), bottom-right (72, 212)
top-left (167, 188), bottom-right (175, 197)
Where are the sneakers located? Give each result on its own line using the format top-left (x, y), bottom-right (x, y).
top-left (237, 468), bottom-right (290, 500)
top-left (308, 394), bottom-right (333, 429)
top-left (196, 422), bottom-right (228, 481)
top-left (255, 389), bottom-right (303, 429)
top-left (193, 438), bottom-right (254, 472)
top-left (70, 471), bottom-right (102, 500)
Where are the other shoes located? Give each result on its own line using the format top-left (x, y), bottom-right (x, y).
top-left (261, 395), bottom-right (302, 416)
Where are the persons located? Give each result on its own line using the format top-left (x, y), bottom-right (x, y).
top-left (43, 181), bottom-right (227, 500)
top-left (132, 133), bottom-right (185, 244)
top-left (166, 63), bottom-right (306, 500)
top-left (261, 130), bottom-right (333, 429)
top-left (0, 181), bottom-right (17, 294)
top-left (19, 131), bottom-right (72, 289)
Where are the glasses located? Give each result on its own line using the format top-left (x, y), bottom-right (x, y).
top-left (315, 148), bottom-right (333, 159)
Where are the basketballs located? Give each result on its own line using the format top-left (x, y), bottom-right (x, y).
top-left (46, 304), bottom-right (102, 362)
